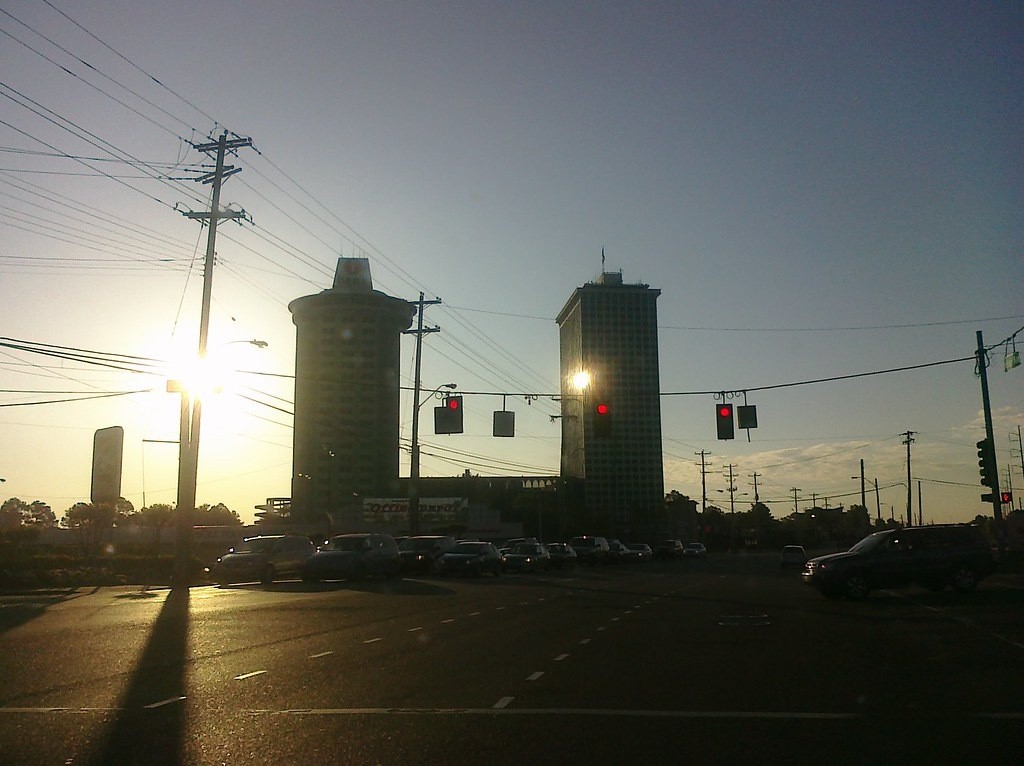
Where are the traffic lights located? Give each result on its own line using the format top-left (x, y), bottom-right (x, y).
top-left (595, 401), bottom-right (611, 437)
top-left (446, 395), bottom-right (463, 435)
top-left (716, 404), bottom-right (736, 440)
top-left (976, 438), bottom-right (991, 488)
top-left (1002, 491), bottom-right (1012, 503)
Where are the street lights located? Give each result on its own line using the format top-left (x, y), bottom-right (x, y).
top-left (851, 474), bottom-right (882, 522)
top-left (410, 384), bottom-right (457, 534)
top-left (178, 340), bottom-right (268, 566)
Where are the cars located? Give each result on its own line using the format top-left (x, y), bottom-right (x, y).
top-left (609, 539), bottom-right (624, 562)
top-left (545, 542), bottom-right (578, 570)
top-left (625, 543), bottom-right (654, 562)
top-left (781, 545), bottom-right (807, 566)
top-left (396, 534), bottom-right (451, 575)
top-left (682, 542), bottom-right (707, 558)
top-left (497, 537), bottom-right (539, 557)
top-left (500, 543), bottom-right (552, 572)
top-left (434, 542), bottom-right (503, 580)
top-left (391, 535), bottom-right (410, 547)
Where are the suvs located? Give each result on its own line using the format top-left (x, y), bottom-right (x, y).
top-left (799, 524), bottom-right (997, 602)
top-left (209, 535), bottom-right (316, 586)
top-left (657, 539), bottom-right (683, 557)
top-left (301, 534), bottom-right (403, 589)
top-left (569, 536), bottom-right (610, 566)
